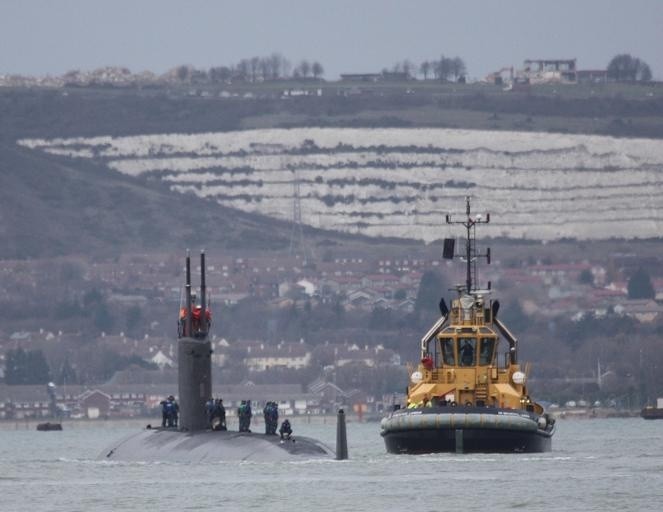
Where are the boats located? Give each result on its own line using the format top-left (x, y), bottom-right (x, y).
top-left (377, 192), bottom-right (555, 455)
top-left (35, 422), bottom-right (62, 431)
top-left (638, 405), bottom-right (662, 419)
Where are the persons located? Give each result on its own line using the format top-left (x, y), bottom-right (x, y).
top-left (460, 340), bottom-right (475, 366)
top-left (158, 394), bottom-right (279, 437)
top-left (278, 418), bottom-right (293, 440)
